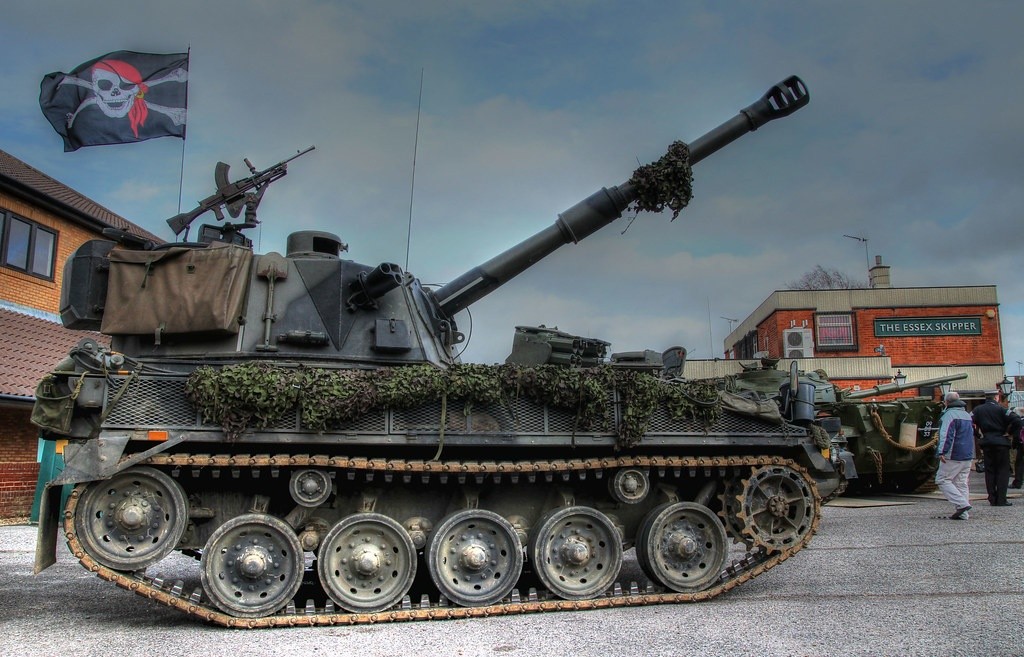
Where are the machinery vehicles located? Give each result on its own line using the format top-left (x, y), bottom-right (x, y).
top-left (840, 372), bottom-right (966, 490)
top-left (31, 71), bottom-right (969, 627)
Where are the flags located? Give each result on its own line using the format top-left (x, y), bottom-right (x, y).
top-left (39, 50), bottom-right (190, 152)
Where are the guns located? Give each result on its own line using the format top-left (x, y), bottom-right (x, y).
top-left (165, 144), bottom-right (317, 246)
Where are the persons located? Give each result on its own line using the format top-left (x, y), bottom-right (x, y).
top-left (935, 392), bottom-right (974, 519)
top-left (998, 393), bottom-right (1008, 407)
top-left (970, 389), bottom-right (1021, 506)
top-left (1008, 406), bottom-right (1024, 489)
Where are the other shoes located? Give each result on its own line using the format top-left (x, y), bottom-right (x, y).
top-left (1008, 480), bottom-right (1022, 489)
top-left (996, 501), bottom-right (1012, 506)
top-left (990, 502), bottom-right (996, 506)
top-left (949, 506), bottom-right (973, 520)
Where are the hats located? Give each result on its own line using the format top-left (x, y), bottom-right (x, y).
top-left (984, 388), bottom-right (1000, 393)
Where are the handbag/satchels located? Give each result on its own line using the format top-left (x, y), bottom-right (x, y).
top-left (975, 459), bottom-right (985, 472)
